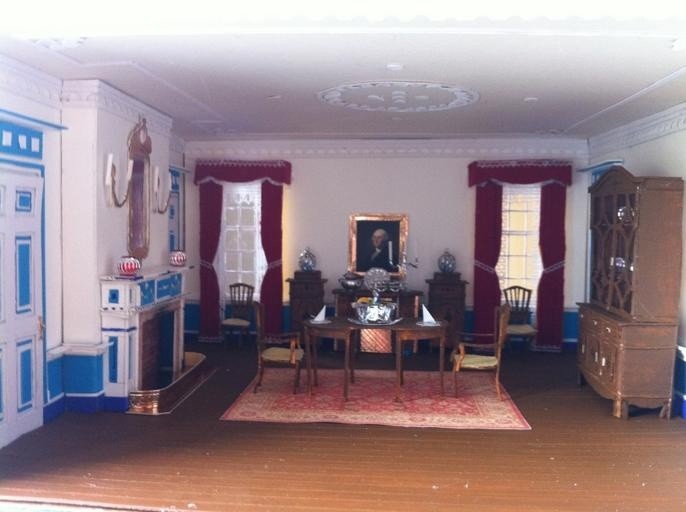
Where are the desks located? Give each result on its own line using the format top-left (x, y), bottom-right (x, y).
top-left (301, 310), bottom-right (451, 400)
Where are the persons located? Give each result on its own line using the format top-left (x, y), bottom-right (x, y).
top-left (365, 228), bottom-right (396, 268)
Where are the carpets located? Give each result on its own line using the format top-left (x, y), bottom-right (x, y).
top-left (217, 362), bottom-right (534, 431)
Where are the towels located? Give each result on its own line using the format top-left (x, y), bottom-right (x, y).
top-left (312, 304), bottom-right (326, 321)
top-left (421, 303), bottom-right (436, 324)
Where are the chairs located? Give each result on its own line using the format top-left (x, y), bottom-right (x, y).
top-left (252, 300), bottom-right (311, 395)
top-left (500, 284), bottom-right (536, 355)
top-left (219, 282), bottom-right (254, 346)
top-left (449, 303), bottom-right (511, 400)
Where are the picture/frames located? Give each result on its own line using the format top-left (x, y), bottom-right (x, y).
top-left (348, 212), bottom-right (409, 280)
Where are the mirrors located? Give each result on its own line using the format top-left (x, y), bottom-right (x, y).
top-left (125, 115), bottom-right (152, 267)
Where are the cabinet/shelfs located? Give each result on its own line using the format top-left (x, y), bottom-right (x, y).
top-left (583, 162), bottom-right (684, 322)
top-left (574, 300), bottom-right (681, 422)
top-left (332, 285), bottom-right (424, 353)
top-left (424, 271), bottom-right (470, 352)
top-left (283, 269), bottom-right (329, 349)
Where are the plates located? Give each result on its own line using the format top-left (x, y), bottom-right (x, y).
top-left (417, 320), bottom-right (441, 327)
top-left (310, 320), bottom-right (333, 325)
